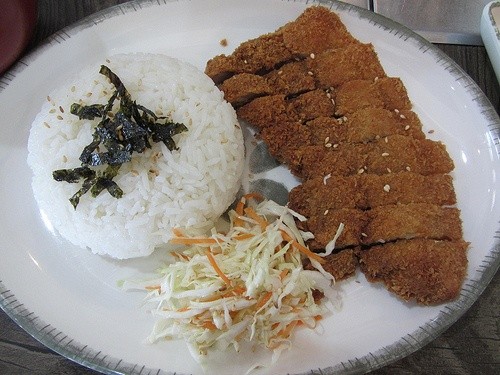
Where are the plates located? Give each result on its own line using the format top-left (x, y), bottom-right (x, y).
top-left (0, 0), bottom-right (500, 375)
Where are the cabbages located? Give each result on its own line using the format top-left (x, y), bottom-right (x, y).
top-left (119, 192), bottom-right (345, 373)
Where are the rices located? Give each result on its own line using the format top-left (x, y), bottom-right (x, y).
top-left (26, 52), bottom-right (244, 259)
